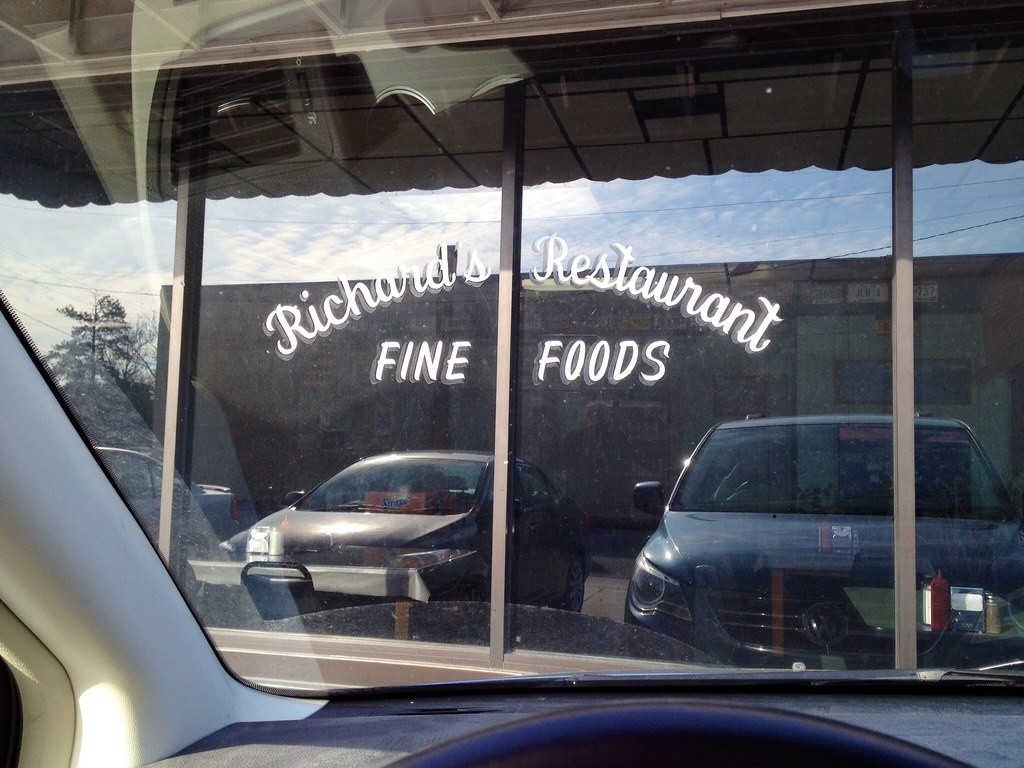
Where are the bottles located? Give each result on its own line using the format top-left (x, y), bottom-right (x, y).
top-left (931, 570), bottom-right (950, 632)
top-left (268, 526), bottom-right (284, 555)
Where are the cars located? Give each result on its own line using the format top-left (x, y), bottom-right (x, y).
top-left (216, 448), bottom-right (594, 632)
top-left (623, 411), bottom-right (1024, 674)
top-left (112, 470), bottom-right (240, 547)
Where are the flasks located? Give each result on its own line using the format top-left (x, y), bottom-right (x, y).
top-left (984, 591), bottom-right (1001, 634)
